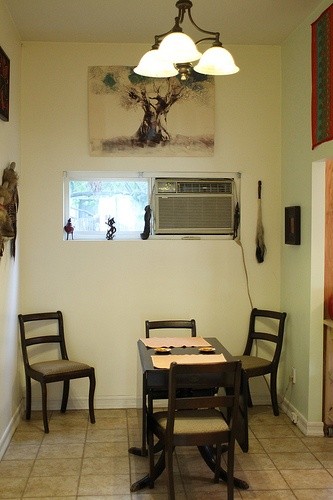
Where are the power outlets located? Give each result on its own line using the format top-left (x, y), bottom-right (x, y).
top-left (290, 364), bottom-right (295, 383)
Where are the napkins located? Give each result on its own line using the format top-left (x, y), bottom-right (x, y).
top-left (150, 353), bottom-right (227, 369)
top-left (139, 337), bottom-right (212, 349)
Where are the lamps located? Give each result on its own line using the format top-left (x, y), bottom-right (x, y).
top-left (133, 0), bottom-right (241, 80)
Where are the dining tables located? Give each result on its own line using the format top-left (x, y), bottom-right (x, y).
top-left (129, 337), bottom-right (250, 493)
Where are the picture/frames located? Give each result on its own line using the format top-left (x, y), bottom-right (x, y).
top-left (0, 45), bottom-right (11, 123)
top-left (284, 205), bottom-right (302, 245)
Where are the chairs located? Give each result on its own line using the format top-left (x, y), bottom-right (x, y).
top-left (234, 307), bottom-right (288, 418)
top-left (150, 359), bottom-right (241, 500)
top-left (145, 319), bottom-right (196, 338)
top-left (18, 311), bottom-right (96, 435)
